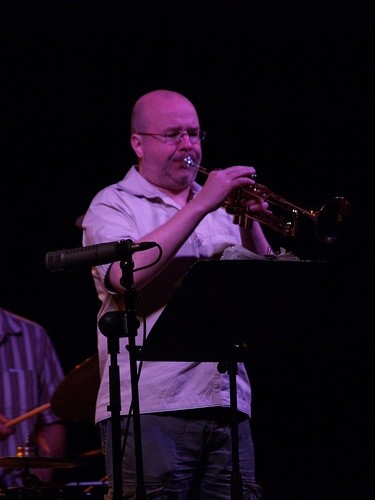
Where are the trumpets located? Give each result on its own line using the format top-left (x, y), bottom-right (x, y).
top-left (182, 156), bottom-right (353, 247)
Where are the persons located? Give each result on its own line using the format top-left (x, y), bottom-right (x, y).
top-left (0, 308), bottom-right (67, 488)
top-left (81, 89), bottom-right (275, 500)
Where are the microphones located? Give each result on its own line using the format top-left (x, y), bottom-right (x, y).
top-left (46, 239), bottom-right (156, 270)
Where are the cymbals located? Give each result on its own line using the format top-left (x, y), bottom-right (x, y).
top-left (50, 352), bottom-right (101, 423)
top-left (0, 456), bottom-right (74, 468)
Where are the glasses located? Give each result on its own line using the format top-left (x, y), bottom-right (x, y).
top-left (138, 129), bottom-right (206, 144)
